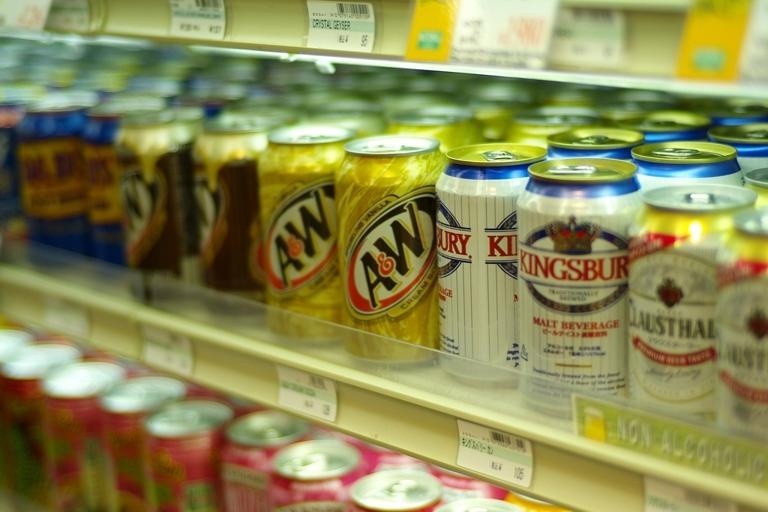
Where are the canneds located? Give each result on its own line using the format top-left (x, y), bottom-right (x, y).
top-left (0, 41), bottom-right (768, 512)
